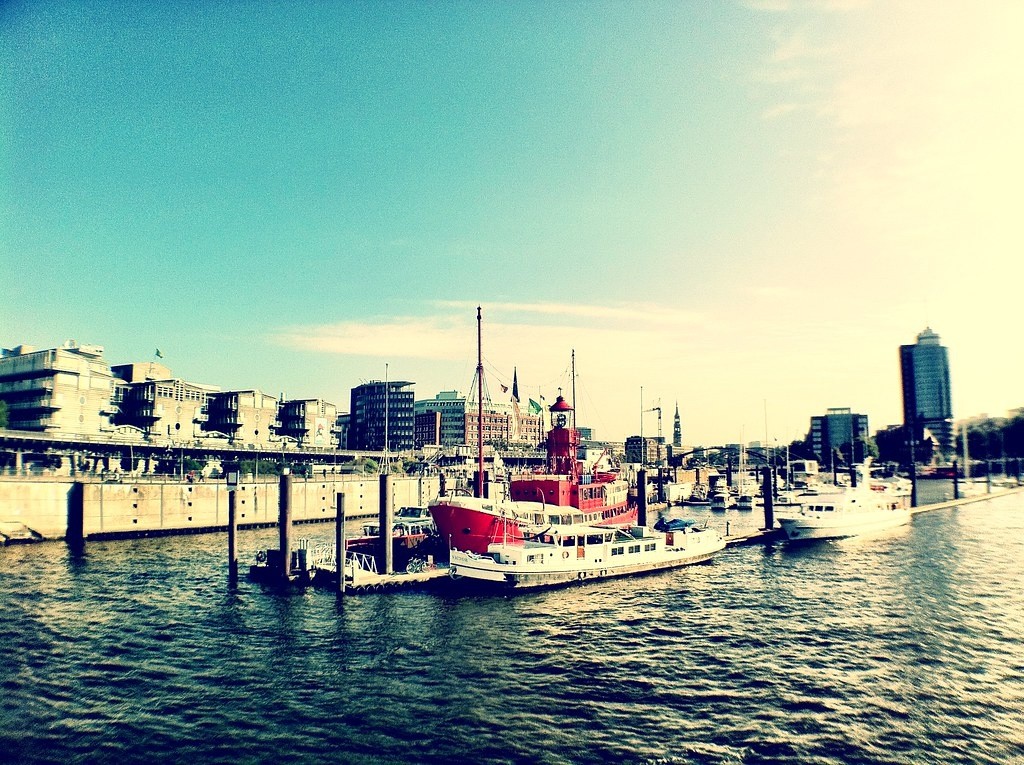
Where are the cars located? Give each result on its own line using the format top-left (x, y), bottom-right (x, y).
top-left (155, 457), bottom-right (204, 476)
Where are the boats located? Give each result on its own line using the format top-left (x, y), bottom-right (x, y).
top-left (674, 480), bottom-right (794, 510)
top-left (426, 483), bottom-right (728, 590)
top-left (779, 450), bottom-right (913, 543)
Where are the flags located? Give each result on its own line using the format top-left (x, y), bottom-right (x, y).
top-left (528, 398), bottom-right (542, 415)
top-left (156, 348), bottom-right (164, 358)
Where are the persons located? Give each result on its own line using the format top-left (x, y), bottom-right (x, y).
top-left (187, 470), bottom-right (196, 483)
top-left (323, 469), bottom-right (326, 477)
top-left (331, 467), bottom-right (334, 474)
top-left (305, 470), bottom-right (309, 481)
top-left (114, 468), bottom-right (117, 472)
top-left (198, 470), bottom-right (204, 482)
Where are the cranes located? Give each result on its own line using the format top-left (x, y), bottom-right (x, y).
top-left (643, 397), bottom-right (662, 436)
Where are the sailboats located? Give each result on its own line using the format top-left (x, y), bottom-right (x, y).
top-left (350, 303), bottom-right (650, 566)
